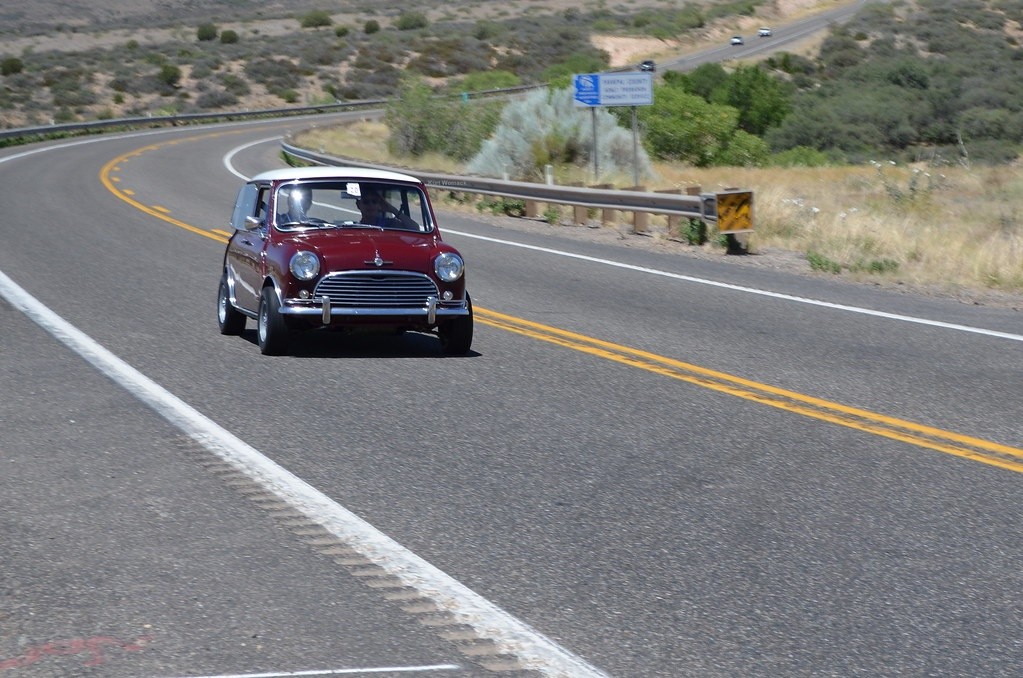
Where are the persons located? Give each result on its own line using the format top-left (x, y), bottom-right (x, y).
top-left (259, 187), bottom-right (328, 229)
top-left (355, 189), bottom-right (421, 231)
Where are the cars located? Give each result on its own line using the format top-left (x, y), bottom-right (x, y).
top-left (214, 166), bottom-right (474, 358)
top-left (731, 36), bottom-right (744, 46)
top-left (640, 61), bottom-right (655, 72)
top-left (759, 26), bottom-right (773, 38)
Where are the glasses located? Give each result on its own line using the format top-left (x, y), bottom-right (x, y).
top-left (360, 199), bottom-right (379, 205)
